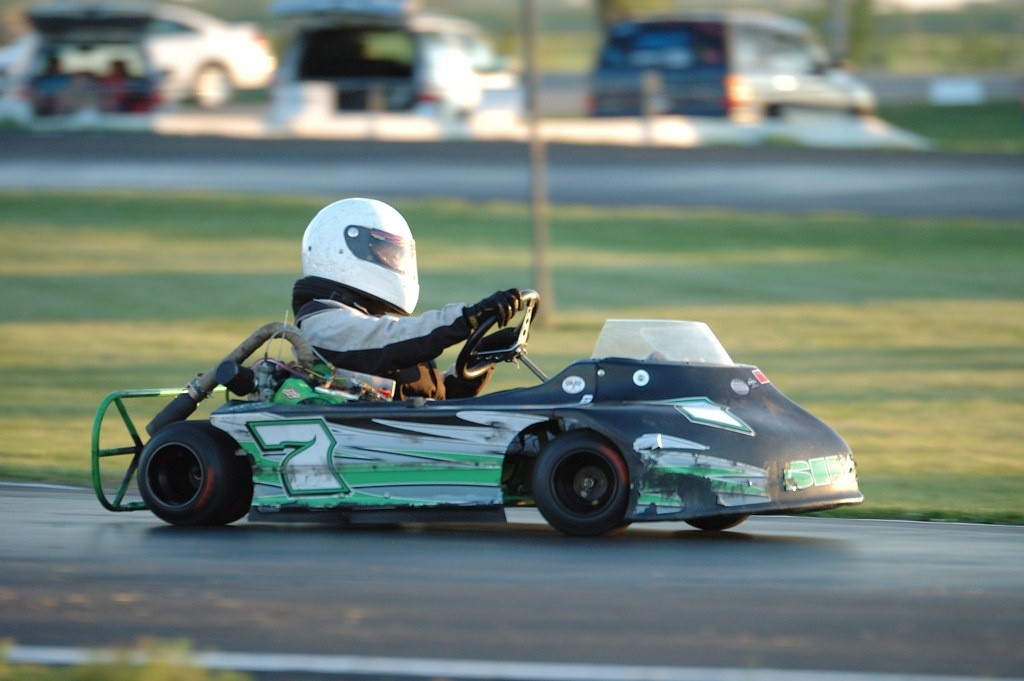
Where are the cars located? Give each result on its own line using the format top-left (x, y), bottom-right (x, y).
top-left (1, 0), bottom-right (275, 115)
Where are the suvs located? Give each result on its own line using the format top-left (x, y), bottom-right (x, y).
top-left (278, 0), bottom-right (523, 133)
top-left (588, 10), bottom-right (881, 119)
top-left (6, 2), bottom-right (176, 119)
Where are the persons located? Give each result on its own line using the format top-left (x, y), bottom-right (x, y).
top-left (291, 198), bottom-right (525, 402)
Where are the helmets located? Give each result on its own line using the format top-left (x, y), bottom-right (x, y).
top-left (301, 197), bottom-right (420, 316)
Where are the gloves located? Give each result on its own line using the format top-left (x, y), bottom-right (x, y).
top-left (467, 287), bottom-right (524, 329)
top-left (472, 326), bottom-right (530, 360)
